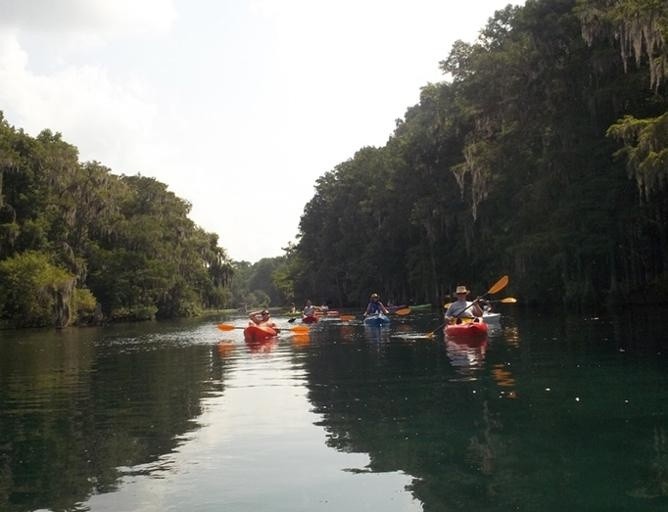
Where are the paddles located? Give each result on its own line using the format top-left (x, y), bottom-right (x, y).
top-left (444, 297), bottom-right (517, 309)
top-left (340, 308), bottom-right (411, 320)
top-left (288, 307), bottom-right (314, 323)
top-left (426, 275), bottom-right (509, 338)
top-left (217, 324), bottom-right (310, 335)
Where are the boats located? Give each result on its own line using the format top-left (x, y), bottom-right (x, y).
top-left (303, 316), bottom-right (319, 324)
top-left (445, 321), bottom-right (487, 337)
top-left (318, 310), bottom-right (338, 316)
top-left (363, 316), bottom-right (389, 325)
top-left (483, 312), bottom-right (501, 324)
top-left (244, 324), bottom-right (275, 338)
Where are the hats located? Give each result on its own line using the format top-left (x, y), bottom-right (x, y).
top-left (453, 286), bottom-right (470, 298)
top-left (370, 293), bottom-right (380, 301)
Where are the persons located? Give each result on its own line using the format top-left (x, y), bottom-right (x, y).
top-left (290, 302), bottom-right (296, 313)
top-left (362, 293), bottom-right (390, 317)
top-left (301, 299), bottom-right (323, 319)
top-left (443, 285), bottom-right (484, 325)
top-left (249, 309), bottom-right (281, 334)
top-left (478, 298), bottom-right (494, 312)
